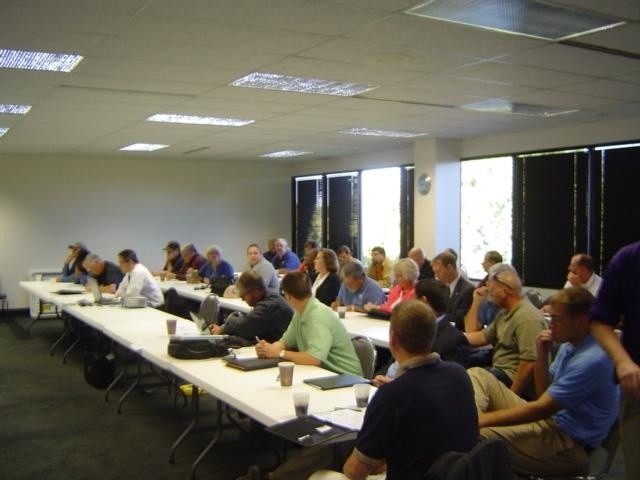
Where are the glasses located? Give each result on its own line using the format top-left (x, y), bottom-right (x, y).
top-left (488, 271), bottom-right (513, 291)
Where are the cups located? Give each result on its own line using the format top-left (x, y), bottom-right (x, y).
top-left (278, 362), bottom-right (295, 387)
top-left (354, 383), bottom-right (370, 407)
top-left (159, 272), bottom-right (166, 281)
top-left (336, 305), bottom-right (346, 318)
top-left (166, 318), bottom-right (177, 334)
top-left (291, 390), bottom-right (310, 416)
top-left (186, 274), bottom-right (192, 284)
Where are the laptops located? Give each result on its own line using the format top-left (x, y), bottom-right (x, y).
top-left (90, 278), bottom-right (120, 305)
top-left (189, 310), bottom-right (213, 335)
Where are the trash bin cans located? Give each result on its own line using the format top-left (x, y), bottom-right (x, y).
top-left (82, 347), bottom-right (116, 389)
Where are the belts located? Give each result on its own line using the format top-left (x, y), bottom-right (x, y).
top-left (569, 434), bottom-right (594, 455)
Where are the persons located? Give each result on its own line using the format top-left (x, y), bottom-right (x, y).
top-left (588, 241), bottom-right (640, 402)
top-left (210, 236), bottom-right (622, 480)
top-left (61, 241), bottom-right (234, 361)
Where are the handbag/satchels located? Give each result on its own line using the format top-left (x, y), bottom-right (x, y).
top-left (194, 276), bottom-right (234, 297)
top-left (168, 334), bottom-right (230, 359)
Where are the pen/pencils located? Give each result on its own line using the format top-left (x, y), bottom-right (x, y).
top-left (255, 335), bottom-right (260, 342)
top-left (335, 406), bottom-right (362, 412)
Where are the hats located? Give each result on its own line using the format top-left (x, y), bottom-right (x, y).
top-left (68, 243), bottom-right (87, 251)
top-left (162, 242), bottom-right (179, 251)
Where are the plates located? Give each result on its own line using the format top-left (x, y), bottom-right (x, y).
top-left (123, 295), bottom-right (147, 308)
